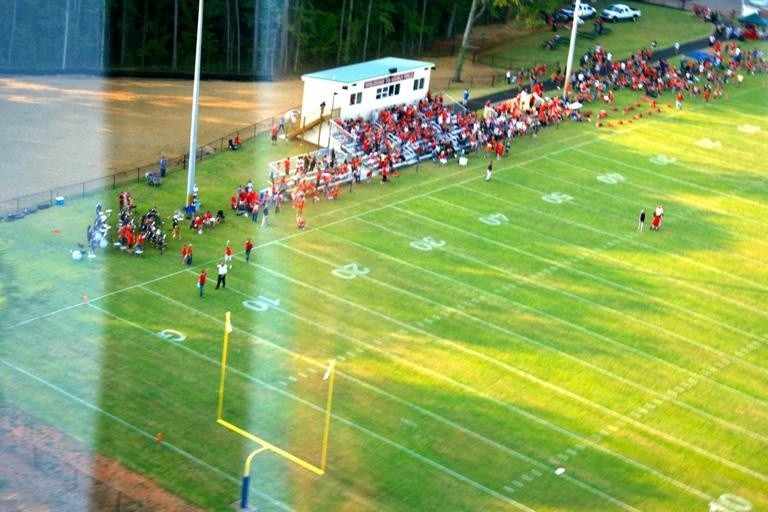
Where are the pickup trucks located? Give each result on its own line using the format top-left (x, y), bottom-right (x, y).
top-left (600, 4), bottom-right (642, 23)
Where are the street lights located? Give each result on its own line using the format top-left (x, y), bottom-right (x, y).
top-left (315, 100), bottom-right (325, 151)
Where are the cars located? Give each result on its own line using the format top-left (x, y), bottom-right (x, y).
top-left (744, 0), bottom-right (766, 8)
top-left (559, 2), bottom-right (597, 23)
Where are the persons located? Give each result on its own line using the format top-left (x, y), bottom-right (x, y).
top-left (551, 44), bottom-right (620, 104)
top-left (235, 132), bottom-right (242, 147)
top-left (245, 238), bottom-right (253, 260)
top-left (229, 135), bottom-right (236, 150)
top-left (506, 63), bottom-right (547, 84)
top-left (336, 116), bottom-right (406, 185)
top-left (483, 82), bottom-right (582, 182)
top-left (374, 88), bottom-right (488, 168)
top-left (261, 147), bottom-right (361, 229)
top-left (638, 210), bottom-right (645, 231)
top-left (198, 270), bottom-right (207, 297)
top-left (215, 263), bottom-right (230, 289)
top-left (86, 186), bottom-right (224, 267)
top-left (692, 4), bottom-right (762, 47)
top-left (712, 39), bottom-right (763, 99)
top-left (650, 205), bottom-right (663, 230)
top-left (279, 116), bottom-right (286, 133)
top-left (231, 180), bottom-right (262, 218)
top-left (271, 126), bottom-right (279, 144)
top-left (225, 240), bottom-right (233, 261)
top-left (159, 156), bottom-right (168, 178)
top-left (597, 17), bottom-right (605, 35)
top-left (617, 47), bottom-right (715, 111)
top-left (548, 16), bottom-right (558, 33)
